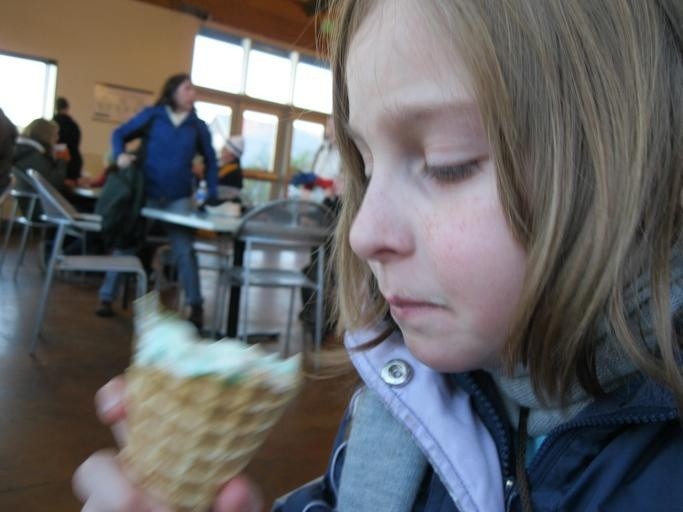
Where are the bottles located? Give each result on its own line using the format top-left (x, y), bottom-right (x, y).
top-left (196, 180), bottom-right (208, 208)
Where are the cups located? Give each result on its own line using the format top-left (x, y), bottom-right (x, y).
top-left (51, 143), bottom-right (66, 161)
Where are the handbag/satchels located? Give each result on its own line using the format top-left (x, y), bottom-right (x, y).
top-left (95, 161), bottom-right (146, 246)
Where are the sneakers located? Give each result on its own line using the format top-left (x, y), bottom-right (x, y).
top-left (95, 299), bottom-right (113, 317)
top-left (188, 304), bottom-right (204, 327)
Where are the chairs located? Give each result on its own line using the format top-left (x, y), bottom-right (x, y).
top-left (2, 164), bottom-right (86, 285)
top-left (149, 185), bottom-right (256, 337)
top-left (212, 197), bottom-right (338, 374)
top-left (26, 168), bottom-right (146, 357)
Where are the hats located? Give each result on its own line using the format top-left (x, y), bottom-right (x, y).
top-left (224, 136), bottom-right (245, 160)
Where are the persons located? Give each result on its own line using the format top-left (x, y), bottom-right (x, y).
top-left (1, 73), bottom-right (344, 322)
top-left (73, 0), bottom-right (683, 510)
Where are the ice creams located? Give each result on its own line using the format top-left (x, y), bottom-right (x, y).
top-left (115, 292), bottom-right (302, 510)
